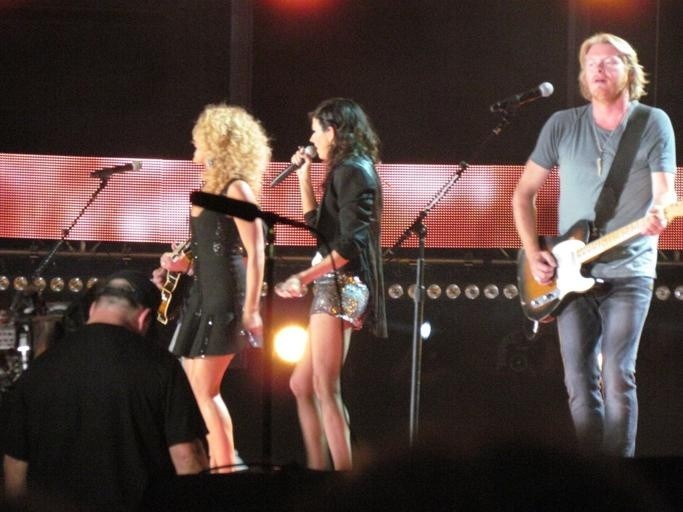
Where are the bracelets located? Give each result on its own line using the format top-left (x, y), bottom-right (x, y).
top-left (185, 259), bottom-right (191, 275)
top-left (241, 306), bottom-right (261, 315)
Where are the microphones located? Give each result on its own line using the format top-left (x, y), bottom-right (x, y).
top-left (90, 159), bottom-right (145, 178)
top-left (491, 81), bottom-right (555, 113)
top-left (270, 143), bottom-right (317, 187)
top-left (187, 188), bottom-right (279, 226)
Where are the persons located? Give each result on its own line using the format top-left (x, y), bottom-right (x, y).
top-left (1, 274), bottom-right (210, 504)
top-left (164, 101), bottom-right (274, 474)
top-left (281, 97), bottom-right (389, 473)
top-left (512, 31), bottom-right (678, 458)
top-left (151, 240), bottom-right (198, 293)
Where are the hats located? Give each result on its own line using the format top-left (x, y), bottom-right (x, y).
top-left (91, 270), bottom-right (163, 336)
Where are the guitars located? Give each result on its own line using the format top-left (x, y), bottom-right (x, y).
top-left (516, 200), bottom-right (683, 324)
top-left (157, 240), bottom-right (191, 323)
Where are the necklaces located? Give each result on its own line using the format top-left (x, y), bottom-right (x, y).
top-left (591, 105), bottom-right (632, 178)
